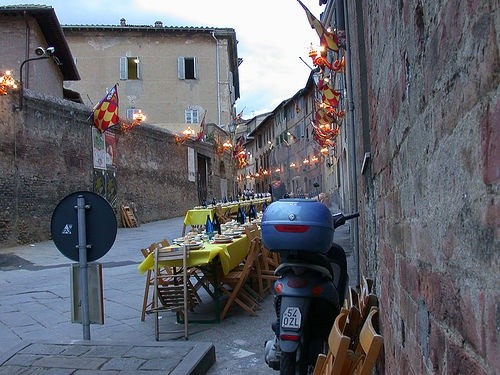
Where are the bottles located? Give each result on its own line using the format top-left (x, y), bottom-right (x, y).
top-left (246, 190), bottom-right (250, 201)
top-left (262, 200), bottom-right (268, 213)
top-left (212, 197), bottom-right (215, 206)
top-left (236, 204), bottom-right (244, 225)
top-left (249, 202), bottom-right (256, 222)
top-left (206, 214), bottom-right (213, 235)
top-left (212, 213), bottom-right (221, 234)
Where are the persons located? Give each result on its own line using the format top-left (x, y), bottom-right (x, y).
top-left (244, 189), bottom-right (254, 196)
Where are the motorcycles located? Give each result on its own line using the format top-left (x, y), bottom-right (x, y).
top-left (260, 198), bottom-right (360, 375)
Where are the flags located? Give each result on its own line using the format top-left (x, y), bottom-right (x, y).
top-left (316, 79), bottom-right (340, 109)
top-left (300, 4), bottom-right (338, 53)
top-left (193, 117), bottom-right (205, 143)
top-left (90, 85), bottom-right (121, 134)
top-left (311, 103), bottom-right (343, 149)
top-left (233, 136), bottom-right (247, 169)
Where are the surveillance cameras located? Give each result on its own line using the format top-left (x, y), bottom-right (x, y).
top-left (47, 47), bottom-right (54, 54)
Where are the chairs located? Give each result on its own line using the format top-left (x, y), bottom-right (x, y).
top-left (141, 244), bottom-right (194, 321)
top-left (245, 223), bottom-right (281, 298)
top-left (312, 275), bottom-right (384, 375)
top-left (157, 239), bottom-right (213, 302)
top-left (144, 245), bottom-right (188, 341)
top-left (216, 236), bottom-right (263, 320)
top-left (121, 205), bottom-right (138, 228)
top-left (216, 204), bottom-right (238, 224)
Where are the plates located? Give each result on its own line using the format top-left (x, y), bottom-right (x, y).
top-left (172, 237), bottom-right (196, 243)
top-left (225, 230), bottom-right (243, 238)
top-left (214, 234), bottom-right (233, 244)
top-left (180, 241), bottom-right (204, 250)
